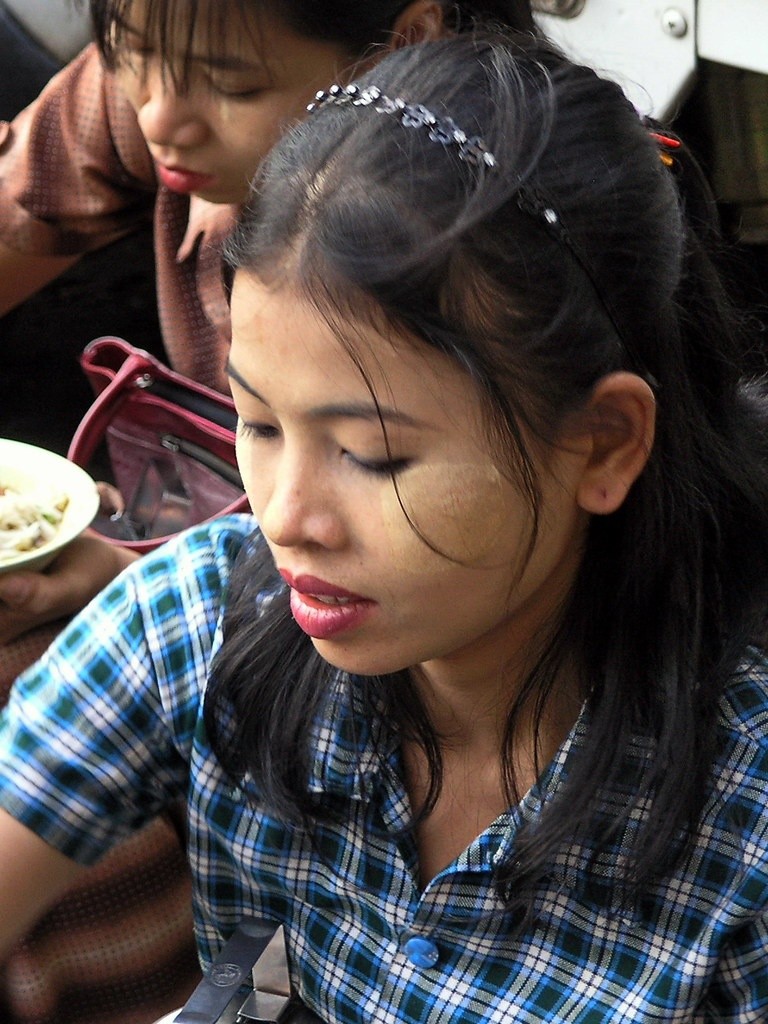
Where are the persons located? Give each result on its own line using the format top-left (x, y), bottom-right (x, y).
top-left (0, 27), bottom-right (768, 1024)
top-left (0, 0), bottom-right (512, 1024)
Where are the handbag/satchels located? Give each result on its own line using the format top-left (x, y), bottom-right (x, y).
top-left (65, 336), bottom-right (254, 556)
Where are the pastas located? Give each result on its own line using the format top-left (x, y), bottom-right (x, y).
top-left (0, 471), bottom-right (58, 564)
